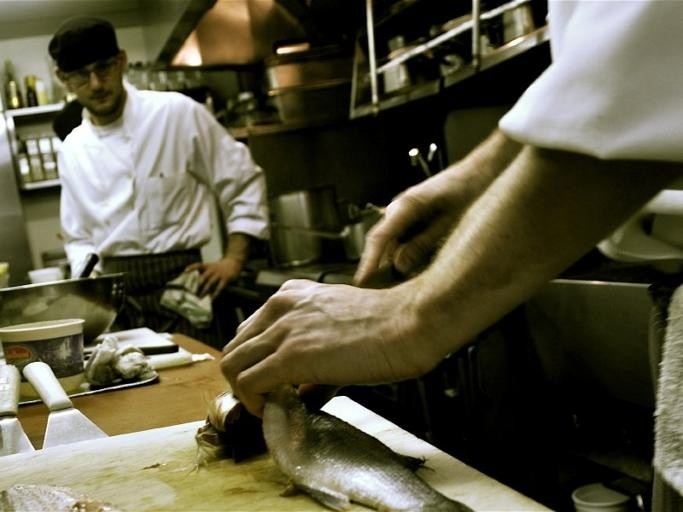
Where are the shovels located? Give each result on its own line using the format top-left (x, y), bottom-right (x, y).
top-left (0, 362), bottom-right (110, 456)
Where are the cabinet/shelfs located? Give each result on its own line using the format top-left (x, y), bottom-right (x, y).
top-left (3, 86), bottom-right (226, 192)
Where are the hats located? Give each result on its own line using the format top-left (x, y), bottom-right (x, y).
top-left (48, 15), bottom-right (120, 73)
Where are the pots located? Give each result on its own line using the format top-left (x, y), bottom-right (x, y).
top-left (213, 39), bottom-right (339, 128)
top-left (267, 186), bottom-right (388, 273)
top-left (0, 252), bottom-right (132, 347)
top-left (377, 0), bottom-right (548, 95)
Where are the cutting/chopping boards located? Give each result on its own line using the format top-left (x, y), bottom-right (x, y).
top-left (84, 324), bottom-right (193, 373)
top-left (0, 391), bottom-right (557, 512)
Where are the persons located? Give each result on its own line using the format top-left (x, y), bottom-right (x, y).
top-left (222, 0), bottom-right (683, 512)
top-left (48, 16), bottom-right (270, 346)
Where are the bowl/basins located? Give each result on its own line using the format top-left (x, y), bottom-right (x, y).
top-left (0, 316), bottom-right (87, 398)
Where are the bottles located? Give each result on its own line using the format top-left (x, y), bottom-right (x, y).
top-left (2, 59), bottom-right (48, 110)
top-left (128, 60), bottom-right (210, 91)
top-left (16, 133), bottom-right (64, 185)
